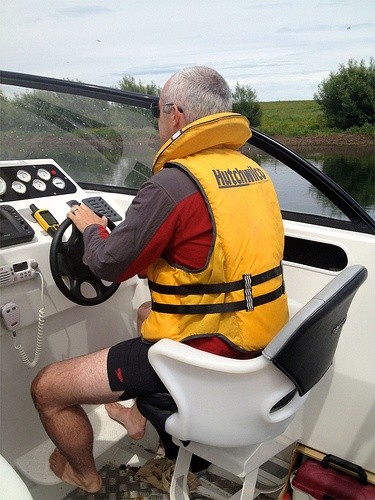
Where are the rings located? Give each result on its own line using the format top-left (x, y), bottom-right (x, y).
top-left (73, 207), bottom-right (78, 214)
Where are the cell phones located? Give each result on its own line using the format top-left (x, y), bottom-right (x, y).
top-left (30, 204), bottom-right (61, 238)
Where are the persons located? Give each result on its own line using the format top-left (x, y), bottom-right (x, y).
top-left (29, 67), bottom-right (289, 494)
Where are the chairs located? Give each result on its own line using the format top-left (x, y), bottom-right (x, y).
top-left (147, 265), bottom-right (368, 500)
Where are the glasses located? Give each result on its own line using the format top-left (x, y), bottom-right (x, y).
top-left (150, 102), bottom-right (184, 118)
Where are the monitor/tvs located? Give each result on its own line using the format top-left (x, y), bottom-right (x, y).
top-left (0, 205), bottom-right (35, 247)
